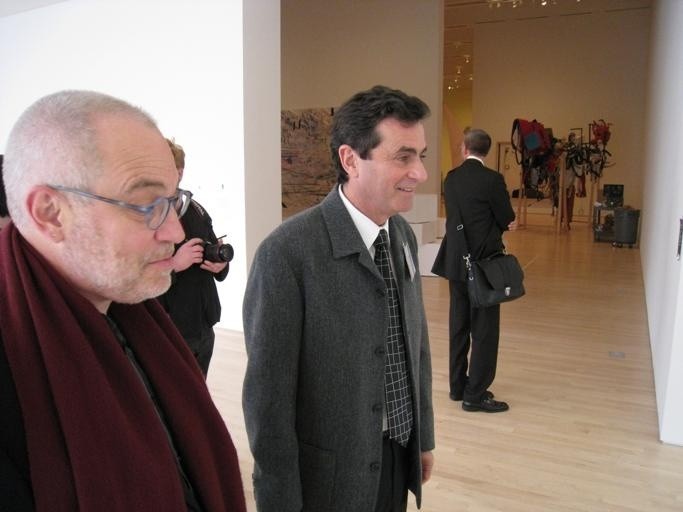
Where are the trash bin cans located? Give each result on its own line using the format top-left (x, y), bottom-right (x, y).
top-left (612, 205), bottom-right (640, 248)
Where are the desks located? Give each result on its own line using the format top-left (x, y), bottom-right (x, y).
top-left (593, 206), bottom-right (619, 242)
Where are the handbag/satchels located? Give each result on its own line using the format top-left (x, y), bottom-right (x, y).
top-left (463, 254), bottom-right (527, 311)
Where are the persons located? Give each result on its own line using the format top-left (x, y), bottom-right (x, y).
top-left (152, 136), bottom-right (231, 381)
top-left (239, 84), bottom-right (437, 512)
top-left (430, 127), bottom-right (518, 412)
top-left (0, 89), bottom-right (248, 510)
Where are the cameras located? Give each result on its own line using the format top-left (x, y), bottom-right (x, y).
top-left (178, 238), bottom-right (234, 271)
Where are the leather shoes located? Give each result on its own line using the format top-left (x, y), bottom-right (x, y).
top-left (462, 399), bottom-right (509, 413)
top-left (450, 388), bottom-right (495, 401)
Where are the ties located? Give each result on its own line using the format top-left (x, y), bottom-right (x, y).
top-left (372, 228), bottom-right (415, 449)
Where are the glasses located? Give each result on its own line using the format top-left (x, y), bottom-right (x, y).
top-left (45, 184), bottom-right (193, 230)
